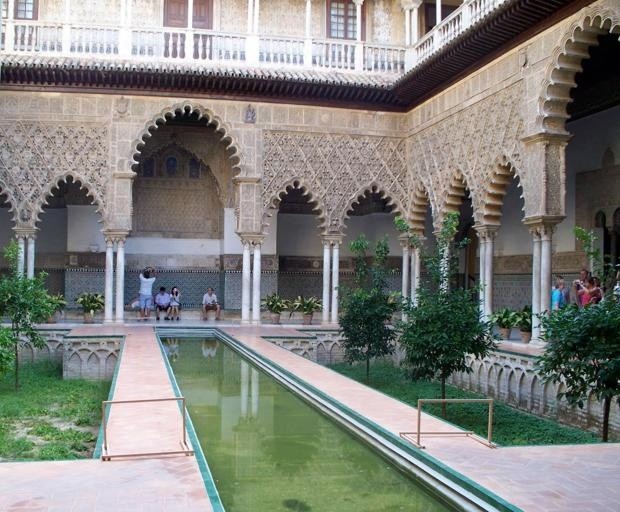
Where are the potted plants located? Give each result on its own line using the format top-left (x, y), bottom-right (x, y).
top-left (289, 295), bottom-right (325, 325)
top-left (73, 292), bottom-right (105, 324)
top-left (484, 308), bottom-right (517, 340)
top-left (383, 290), bottom-right (402, 324)
top-left (45, 292), bottom-right (68, 324)
top-left (260, 293), bottom-right (291, 325)
top-left (508, 305), bottom-right (532, 343)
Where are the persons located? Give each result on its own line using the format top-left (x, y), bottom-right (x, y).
top-left (551, 268), bottom-right (619, 318)
top-left (163, 335), bottom-right (221, 364)
top-left (155, 286), bottom-right (172, 321)
top-left (201, 286), bottom-right (221, 321)
top-left (137, 267), bottom-right (157, 322)
top-left (169, 286), bottom-right (183, 320)
